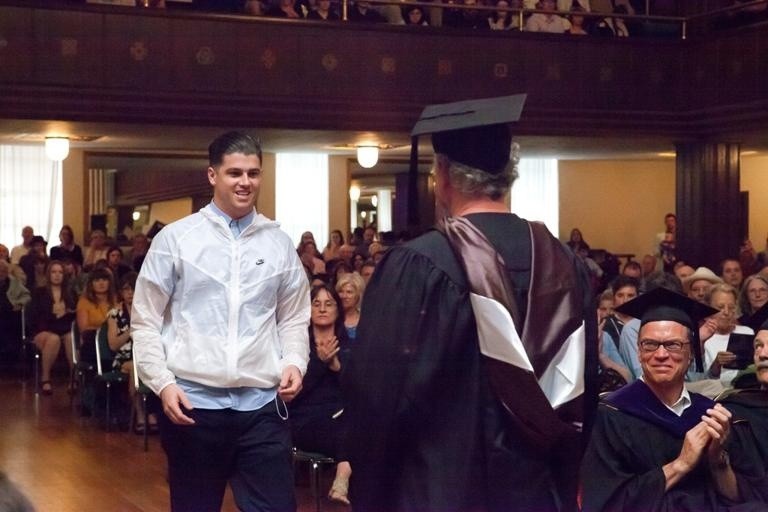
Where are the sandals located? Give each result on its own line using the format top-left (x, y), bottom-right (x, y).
top-left (135, 412), bottom-right (160, 433)
top-left (327, 475), bottom-right (351, 507)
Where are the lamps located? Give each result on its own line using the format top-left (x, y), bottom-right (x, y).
top-left (44, 136), bottom-right (70, 162)
top-left (356, 146), bottom-right (381, 170)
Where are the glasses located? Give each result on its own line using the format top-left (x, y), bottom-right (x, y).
top-left (634, 339), bottom-right (689, 353)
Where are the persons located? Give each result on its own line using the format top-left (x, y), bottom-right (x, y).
top-left (245, 1), bottom-right (639, 38)
top-left (568, 213), bottom-right (767, 512)
top-left (1, 226), bottom-right (160, 432)
top-left (339, 95), bottom-right (598, 512)
top-left (289, 226), bottom-right (408, 507)
top-left (132, 135), bottom-right (311, 512)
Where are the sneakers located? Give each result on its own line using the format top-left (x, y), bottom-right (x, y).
top-left (38, 379), bottom-right (51, 396)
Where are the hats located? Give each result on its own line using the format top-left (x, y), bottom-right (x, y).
top-left (404, 92), bottom-right (530, 232)
top-left (682, 267), bottom-right (724, 287)
top-left (611, 286), bottom-right (721, 327)
